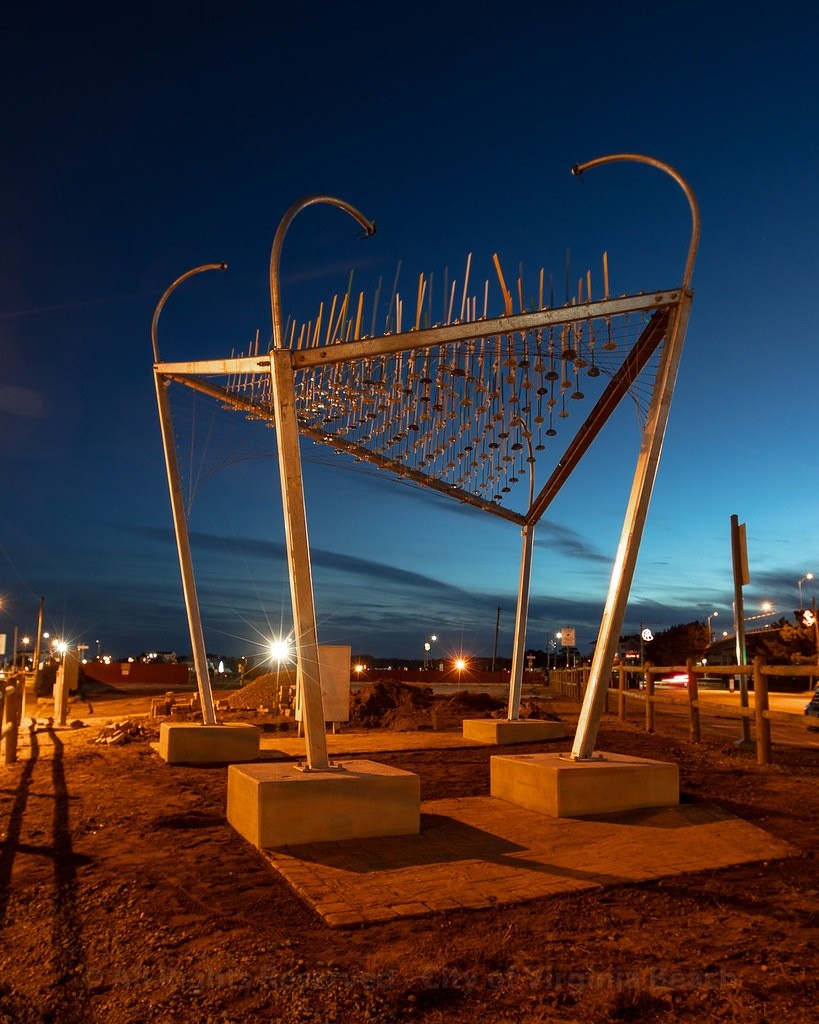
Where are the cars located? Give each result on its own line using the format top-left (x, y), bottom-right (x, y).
top-left (38, 657), bottom-right (51, 670)
top-left (760, 624), bottom-right (772, 630)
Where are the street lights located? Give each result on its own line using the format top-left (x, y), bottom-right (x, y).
top-left (96, 640), bottom-right (100, 659)
top-left (424, 635), bottom-right (437, 667)
top-left (732, 597), bottom-right (745, 634)
top-left (707, 611), bottom-right (719, 643)
top-left (798, 573), bottom-right (813, 610)
top-left (22, 637), bottom-right (29, 672)
